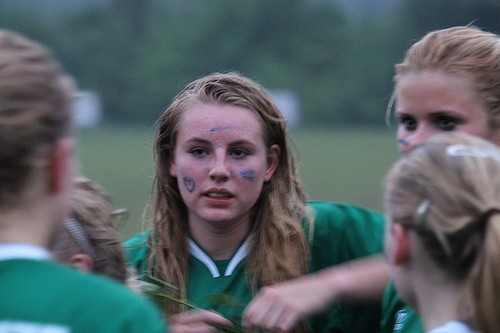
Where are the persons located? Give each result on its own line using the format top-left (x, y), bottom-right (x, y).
top-left (0, 30), bottom-right (169, 333)
top-left (384, 22), bottom-right (500, 333)
top-left (118, 72), bottom-right (393, 333)
